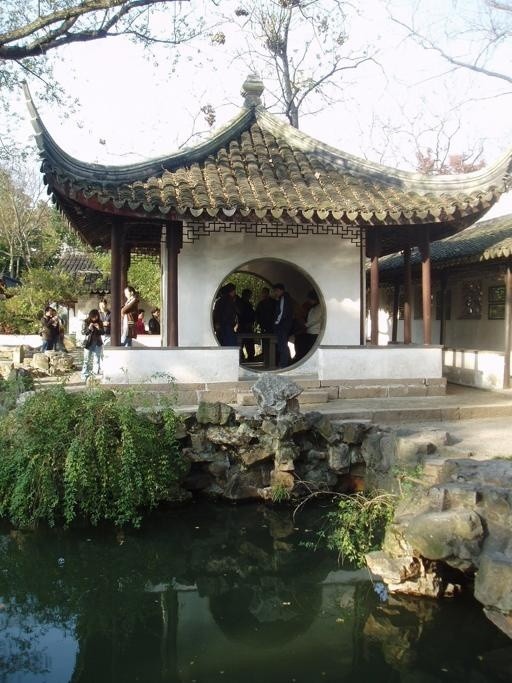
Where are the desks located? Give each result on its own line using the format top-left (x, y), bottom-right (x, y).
top-left (234, 333), bottom-right (277, 368)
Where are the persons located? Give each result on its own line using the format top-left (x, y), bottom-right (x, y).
top-left (96, 298), bottom-right (112, 374)
top-left (137, 308), bottom-right (152, 335)
top-left (50, 309), bottom-right (68, 353)
top-left (149, 307), bottom-right (160, 334)
top-left (212, 282), bottom-right (323, 369)
top-left (80, 308), bottom-right (106, 378)
top-left (34, 306), bottom-right (60, 350)
top-left (120, 285), bottom-right (138, 347)
